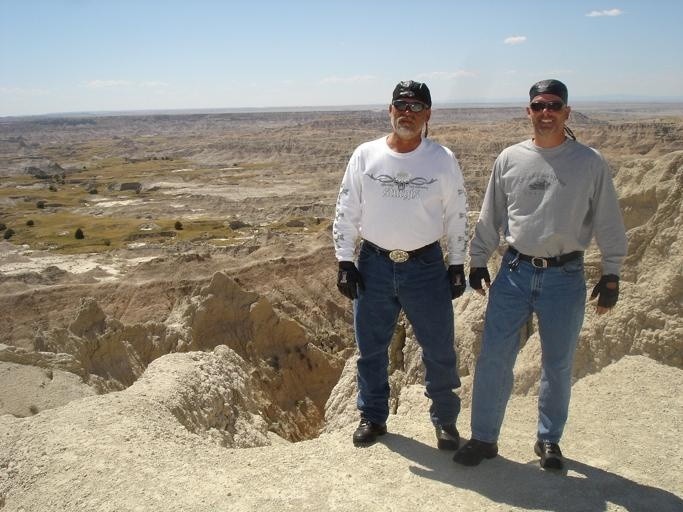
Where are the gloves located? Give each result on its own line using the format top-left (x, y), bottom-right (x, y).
top-left (336, 261), bottom-right (364, 300)
top-left (448, 264), bottom-right (466, 299)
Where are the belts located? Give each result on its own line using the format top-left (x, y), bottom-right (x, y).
top-left (509, 246), bottom-right (584, 270)
top-left (363, 241), bottom-right (437, 263)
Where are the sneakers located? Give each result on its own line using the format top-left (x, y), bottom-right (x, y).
top-left (453, 438), bottom-right (498, 466)
top-left (353, 418), bottom-right (386, 440)
top-left (534, 440), bottom-right (561, 467)
top-left (436, 424), bottom-right (460, 450)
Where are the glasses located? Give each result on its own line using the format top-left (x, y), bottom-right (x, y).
top-left (530, 102), bottom-right (563, 112)
top-left (393, 100), bottom-right (425, 113)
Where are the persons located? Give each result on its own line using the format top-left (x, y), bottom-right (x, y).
top-left (332, 80), bottom-right (468, 449)
top-left (454, 80), bottom-right (629, 469)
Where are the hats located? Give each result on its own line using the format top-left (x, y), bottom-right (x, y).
top-left (529, 79), bottom-right (567, 105)
top-left (393, 81), bottom-right (431, 109)
